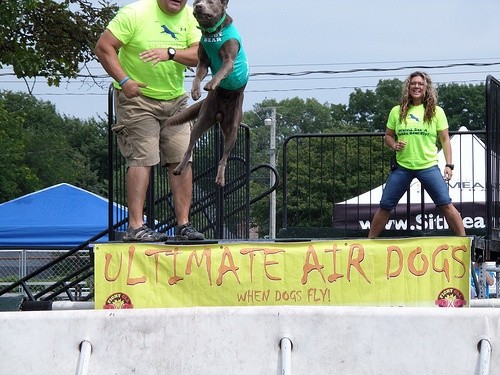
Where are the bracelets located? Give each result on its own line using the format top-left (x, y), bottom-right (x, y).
top-left (119, 76), bottom-right (130, 86)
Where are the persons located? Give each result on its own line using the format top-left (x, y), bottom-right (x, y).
top-left (94, 0), bottom-right (206, 243)
top-left (471, 254), bottom-right (494, 299)
top-left (368, 71), bottom-right (466, 239)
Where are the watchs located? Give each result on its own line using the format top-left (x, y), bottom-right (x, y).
top-left (445, 164), bottom-right (454, 170)
top-left (168, 47), bottom-right (176, 60)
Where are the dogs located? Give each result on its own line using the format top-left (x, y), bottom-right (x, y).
top-left (165, 0), bottom-right (249, 187)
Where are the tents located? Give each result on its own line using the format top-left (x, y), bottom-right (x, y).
top-left (0, 182), bottom-right (175, 253)
top-left (333, 125), bottom-right (500, 237)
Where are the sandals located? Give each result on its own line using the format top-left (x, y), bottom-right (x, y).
top-left (124, 223), bottom-right (169, 242)
top-left (176, 222), bottom-right (206, 240)
